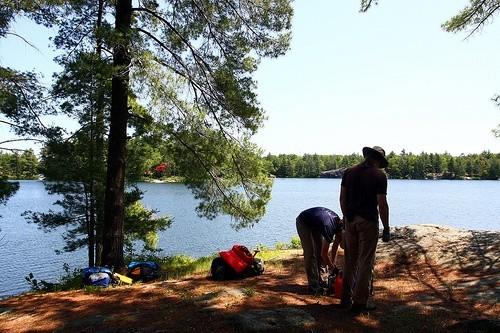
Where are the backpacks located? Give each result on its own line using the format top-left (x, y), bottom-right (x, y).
top-left (80, 266), bottom-right (121, 288)
top-left (126, 260), bottom-right (166, 282)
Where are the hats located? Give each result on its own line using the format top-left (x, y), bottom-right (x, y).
top-left (362, 146), bottom-right (389, 168)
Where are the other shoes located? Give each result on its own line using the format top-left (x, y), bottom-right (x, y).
top-left (354, 302), bottom-right (377, 311)
top-left (340, 302), bottom-right (352, 310)
top-left (309, 290), bottom-right (327, 296)
top-left (320, 279), bottom-right (328, 288)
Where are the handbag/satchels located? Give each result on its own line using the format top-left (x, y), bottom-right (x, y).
top-left (211, 257), bottom-right (266, 279)
top-left (218, 245), bottom-right (256, 272)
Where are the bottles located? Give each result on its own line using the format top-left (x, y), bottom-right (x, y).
top-left (327, 270), bottom-right (344, 299)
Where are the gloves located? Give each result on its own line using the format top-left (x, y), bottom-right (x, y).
top-left (379, 226), bottom-right (391, 241)
top-left (332, 266), bottom-right (340, 276)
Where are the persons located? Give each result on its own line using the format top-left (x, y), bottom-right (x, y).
top-left (296, 207), bottom-right (344, 295)
top-left (339, 146), bottom-right (391, 313)
